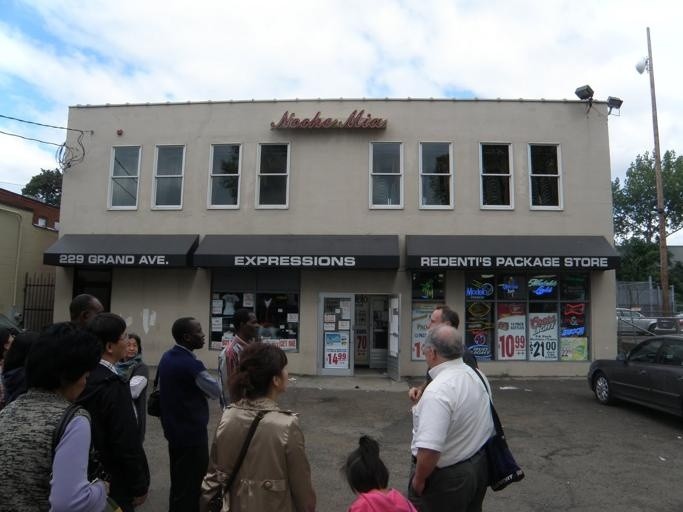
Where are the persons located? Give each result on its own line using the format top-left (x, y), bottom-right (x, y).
top-left (408, 305), bottom-right (481, 403)
top-left (409, 323), bottom-right (497, 512)
top-left (342, 434), bottom-right (418, 512)
top-left (70, 312), bottom-right (151, 511)
top-left (261, 293), bottom-right (275, 324)
top-left (157, 315), bottom-right (219, 512)
top-left (215, 306), bottom-right (261, 414)
top-left (64, 293), bottom-right (105, 323)
top-left (116, 331), bottom-right (148, 448)
top-left (0, 321), bottom-right (111, 512)
top-left (3, 327), bottom-right (44, 411)
top-left (196, 339), bottom-right (316, 512)
top-left (0, 325), bottom-right (20, 407)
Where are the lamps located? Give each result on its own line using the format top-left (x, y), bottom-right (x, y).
top-left (605, 97), bottom-right (623, 116)
top-left (574, 85), bottom-right (593, 116)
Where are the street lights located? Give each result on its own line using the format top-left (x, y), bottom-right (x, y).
top-left (635, 57), bottom-right (669, 316)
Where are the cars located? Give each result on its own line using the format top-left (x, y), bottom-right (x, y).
top-left (616, 307), bottom-right (657, 334)
top-left (587, 334), bottom-right (682, 421)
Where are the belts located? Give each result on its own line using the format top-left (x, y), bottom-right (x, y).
top-left (411, 455), bottom-right (417, 465)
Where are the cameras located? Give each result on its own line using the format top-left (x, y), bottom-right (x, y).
top-left (90, 465), bottom-right (115, 484)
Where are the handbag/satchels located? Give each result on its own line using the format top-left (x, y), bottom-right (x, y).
top-left (88, 449), bottom-right (111, 485)
top-left (206, 486), bottom-right (230, 512)
top-left (487, 434), bottom-right (524, 491)
top-left (148, 390), bottom-right (162, 417)
